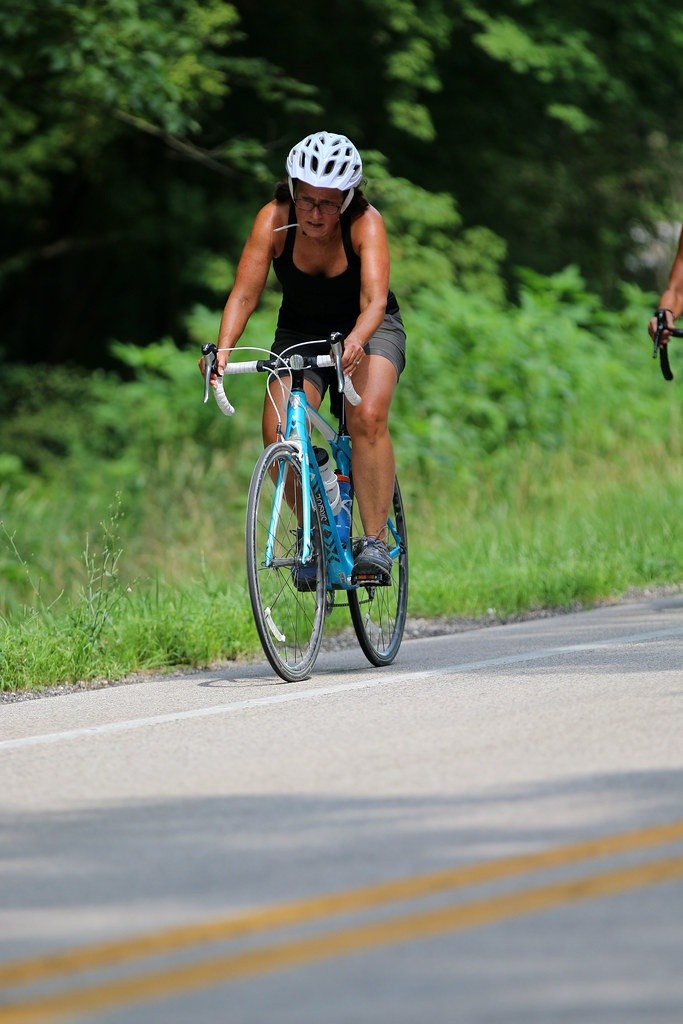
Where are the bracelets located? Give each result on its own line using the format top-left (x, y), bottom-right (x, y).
top-left (657, 309), bottom-right (675, 321)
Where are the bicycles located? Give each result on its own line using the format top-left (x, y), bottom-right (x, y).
top-left (203, 329), bottom-right (411, 683)
top-left (648, 306), bottom-right (683, 381)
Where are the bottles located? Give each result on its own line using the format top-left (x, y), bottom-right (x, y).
top-left (332, 469), bottom-right (352, 543)
top-left (311, 445), bottom-right (342, 517)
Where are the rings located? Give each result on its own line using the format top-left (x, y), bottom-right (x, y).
top-left (357, 361), bottom-right (359, 365)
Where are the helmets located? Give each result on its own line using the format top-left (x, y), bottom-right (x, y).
top-left (285, 131), bottom-right (362, 190)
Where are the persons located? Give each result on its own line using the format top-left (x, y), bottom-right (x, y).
top-left (648, 223), bottom-right (683, 349)
top-left (199, 131), bottom-right (407, 592)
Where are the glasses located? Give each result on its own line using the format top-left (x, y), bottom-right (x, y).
top-left (293, 197), bottom-right (342, 216)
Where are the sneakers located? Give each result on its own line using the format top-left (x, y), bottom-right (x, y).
top-left (352, 527), bottom-right (392, 581)
top-left (292, 526), bottom-right (317, 592)
